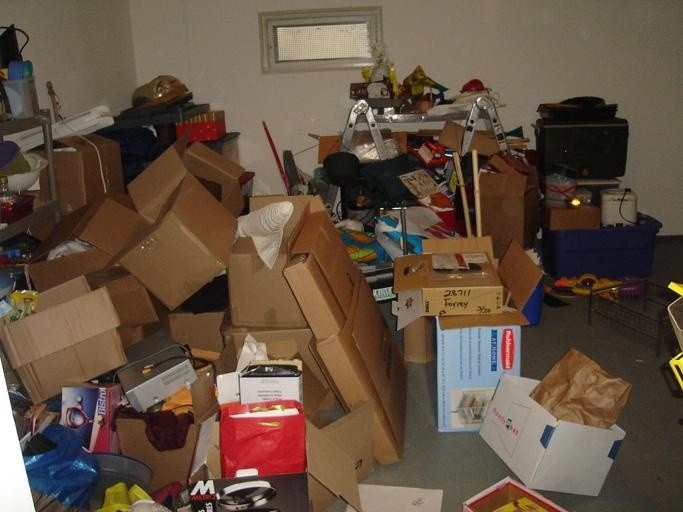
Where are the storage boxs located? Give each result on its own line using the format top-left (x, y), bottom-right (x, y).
top-left (0, 117), bottom-right (663, 512)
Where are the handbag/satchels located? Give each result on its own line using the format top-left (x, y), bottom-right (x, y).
top-left (220, 400), bottom-right (306, 479)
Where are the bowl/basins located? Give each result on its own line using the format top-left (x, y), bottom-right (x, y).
top-left (7, 153), bottom-right (48, 191)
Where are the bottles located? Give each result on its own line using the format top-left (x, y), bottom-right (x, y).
top-left (0, 175), bottom-right (13, 205)
